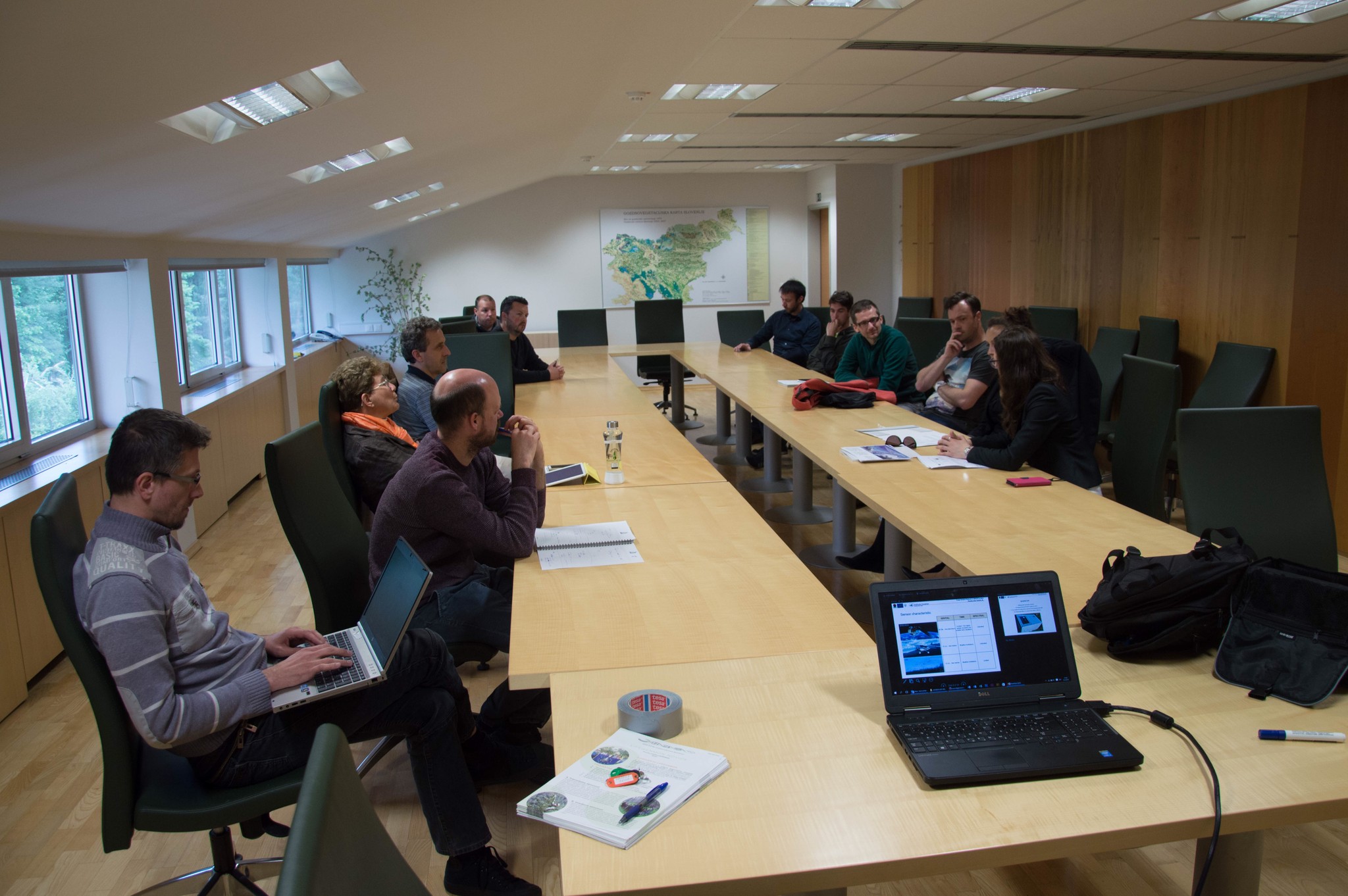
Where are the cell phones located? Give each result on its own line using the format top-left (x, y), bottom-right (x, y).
top-left (547, 464), bottom-right (574, 471)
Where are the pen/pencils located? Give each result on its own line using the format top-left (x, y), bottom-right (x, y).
top-left (618, 782), bottom-right (668, 824)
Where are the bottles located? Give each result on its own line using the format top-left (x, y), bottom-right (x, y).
top-left (603, 420), bottom-right (625, 484)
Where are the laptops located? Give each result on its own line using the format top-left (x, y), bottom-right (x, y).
top-left (266, 535), bottom-right (435, 713)
top-left (869, 570), bottom-right (1143, 786)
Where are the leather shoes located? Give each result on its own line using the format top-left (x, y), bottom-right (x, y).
top-left (900, 566), bottom-right (924, 579)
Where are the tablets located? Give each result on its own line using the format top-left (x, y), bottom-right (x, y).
top-left (545, 462), bottom-right (587, 487)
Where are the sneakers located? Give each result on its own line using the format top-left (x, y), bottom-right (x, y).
top-left (744, 442), bottom-right (788, 468)
top-left (443, 846), bottom-right (542, 896)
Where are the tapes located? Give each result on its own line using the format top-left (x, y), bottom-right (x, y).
top-left (618, 689), bottom-right (683, 741)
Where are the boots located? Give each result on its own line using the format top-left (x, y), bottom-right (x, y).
top-left (835, 516), bottom-right (885, 573)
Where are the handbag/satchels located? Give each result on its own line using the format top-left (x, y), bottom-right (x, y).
top-left (1077, 544), bottom-right (1348, 706)
top-left (821, 390), bottom-right (876, 409)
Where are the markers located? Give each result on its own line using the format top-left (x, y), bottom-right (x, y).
top-left (1258, 729), bottom-right (1345, 743)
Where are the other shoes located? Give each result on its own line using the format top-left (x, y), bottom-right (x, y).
top-left (462, 709), bottom-right (554, 789)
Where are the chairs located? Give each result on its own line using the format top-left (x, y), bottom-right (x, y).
top-left (27, 294), bottom-right (1348, 895)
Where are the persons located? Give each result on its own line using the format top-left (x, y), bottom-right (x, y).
top-left (466, 294), bottom-right (505, 334)
top-left (490, 295), bottom-right (566, 385)
top-left (743, 290), bottom-right (856, 470)
top-left (71, 407), bottom-right (545, 896)
top-left (389, 316), bottom-right (531, 487)
top-left (935, 324), bottom-right (1103, 573)
top-left (329, 356), bottom-right (531, 571)
top-left (826, 299), bottom-right (927, 480)
top-left (899, 304), bottom-right (1106, 587)
top-left (366, 369), bottom-right (548, 737)
top-left (734, 278), bottom-right (822, 445)
top-left (834, 290), bottom-right (999, 577)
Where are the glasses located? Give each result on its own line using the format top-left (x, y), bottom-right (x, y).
top-left (885, 435), bottom-right (916, 449)
top-left (855, 314), bottom-right (880, 327)
top-left (152, 471), bottom-right (202, 489)
top-left (989, 357), bottom-right (999, 365)
top-left (361, 375), bottom-right (390, 407)
